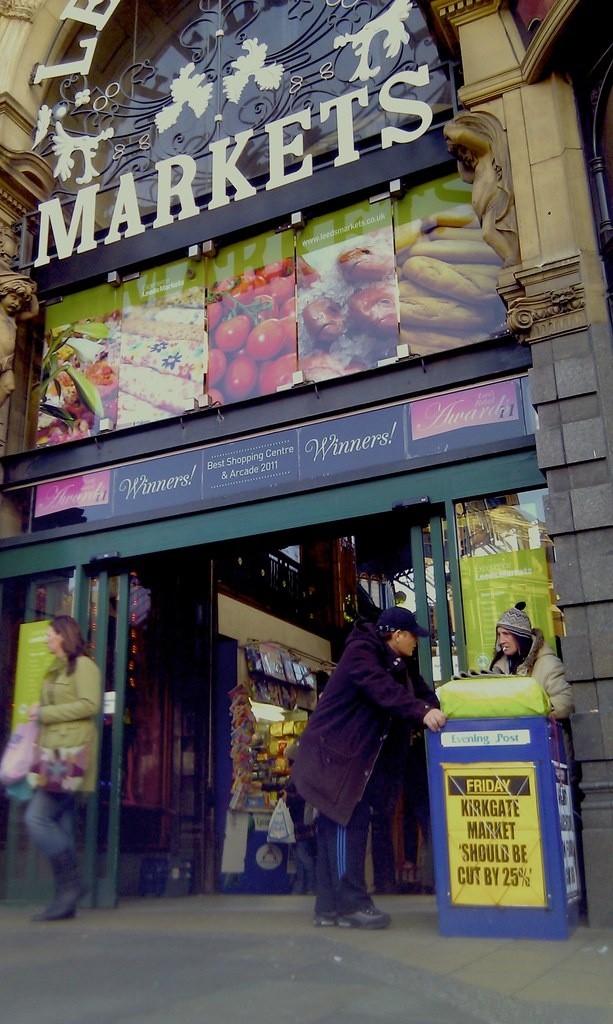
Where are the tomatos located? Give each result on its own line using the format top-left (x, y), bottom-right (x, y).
top-left (207, 258), bottom-right (298, 404)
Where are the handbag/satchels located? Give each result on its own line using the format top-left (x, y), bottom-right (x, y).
top-left (266, 798), bottom-right (296, 844)
top-left (0, 718), bottom-right (38, 783)
top-left (27, 742), bottom-right (90, 793)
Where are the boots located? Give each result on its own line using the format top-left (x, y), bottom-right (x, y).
top-left (31, 850), bottom-right (88, 921)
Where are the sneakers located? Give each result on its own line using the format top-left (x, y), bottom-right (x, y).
top-left (336, 903), bottom-right (390, 930)
top-left (312, 907), bottom-right (337, 925)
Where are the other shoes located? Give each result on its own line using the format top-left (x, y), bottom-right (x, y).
top-left (291, 886), bottom-right (316, 895)
top-left (374, 882), bottom-right (403, 895)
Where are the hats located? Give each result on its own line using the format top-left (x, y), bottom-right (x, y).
top-left (283, 743), bottom-right (298, 760)
top-left (376, 606), bottom-right (429, 638)
top-left (494, 601), bottom-right (531, 652)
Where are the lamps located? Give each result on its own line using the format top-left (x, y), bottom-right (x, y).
top-left (179, 397), bottom-right (199, 428)
top-left (95, 418), bottom-right (113, 447)
top-left (291, 370), bottom-right (319, 398)
top-left (397, 343), bottom-right (426, 373)
top-left (199, 394), bottom-right (224, 421)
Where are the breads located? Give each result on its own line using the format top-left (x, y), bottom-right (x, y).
top-left (396, 208), bottom-right (504, 356)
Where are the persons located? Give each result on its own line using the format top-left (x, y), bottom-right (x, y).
top-left (292, 606), bottom-right (446, 930)
top-left (446, 110), bottom-right (522, 269)
top-left (23, 615), bottom-right (102, 920)
top-left (488, 601), bottom-right (575, 719)
top-left (0, 273), bottom-right (41, 407)
top-left (251, 744), bottom-right (318, 896)
